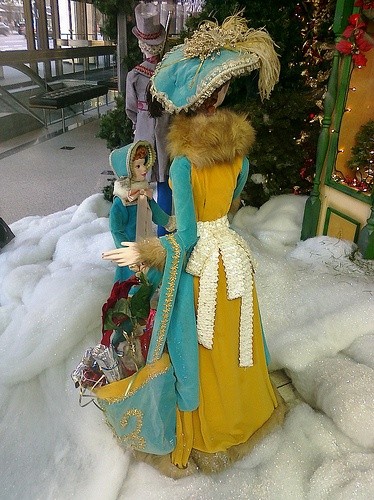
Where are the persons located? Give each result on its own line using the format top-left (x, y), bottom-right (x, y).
top-left (109, 140), bottom-right (177, 299)
top-left (102, 13), bottom-right (284, 470)
top-left (125, 2), bottom-right (179, 239)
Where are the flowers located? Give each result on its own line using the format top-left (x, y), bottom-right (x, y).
top-left (336, 0), bottom-right (374, 66)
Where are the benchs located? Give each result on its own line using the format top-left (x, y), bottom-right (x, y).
top-left (98, 76), bottom-right (118, 98)
top-left (29, 84), bottom-right (109, 134)
